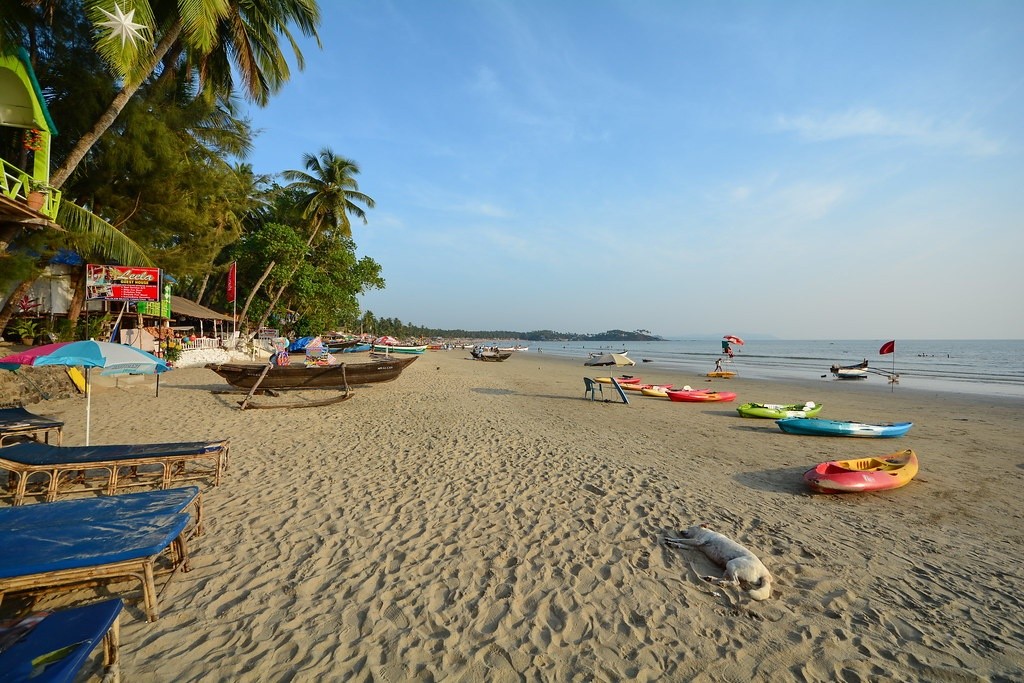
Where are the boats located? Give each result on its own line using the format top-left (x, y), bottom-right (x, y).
top-left (642, 386), bottom-right (710, 397)
top-left (806, 448), bottom-right (921, 494)
top-left (469, 349), bottom-right (512, 363)
top-left (293, 336), bottom-right (445, 354)
top-left (664, 389), bottom-right (736, 404)
top-left (616, 381), bottom-right (673, 392)
top-left (829, 360), bottom-right (872, 378)
top-left (592, 375), bottom-right (640, 385)
top-left (775, 416), bottom-right (915, 439)
top-left (736, 402), bottom-right (822, 420)
top-left (707, 371), bottom-right (736, 378)
top-left (204, 356), bottom-right (420, 388)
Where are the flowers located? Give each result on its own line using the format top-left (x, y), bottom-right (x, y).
top-left (23, 128), bottom-right (45, 151)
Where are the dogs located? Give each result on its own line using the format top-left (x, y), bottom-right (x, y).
top-left (663, 521), bottom-right (773, 600)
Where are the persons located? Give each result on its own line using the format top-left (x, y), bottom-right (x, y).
top-left (918, 353), bottom-right (950, 358)
top-left (714, 358), bottom-right (723, 372)
top-left (443, 341), bottom-right (626, 359)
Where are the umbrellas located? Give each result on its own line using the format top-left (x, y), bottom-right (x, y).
top-left (373, 336), bottom-right (400, 358)
top-left (723, 335), bottom-right (745, 347)
top-left (584, 352), bottom-right (636, 401)
top-left (0, 338), bottom-right (172, 446)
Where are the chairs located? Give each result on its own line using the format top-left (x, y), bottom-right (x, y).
top-left (0, 438), bottom-right (231, 507)
top-left (0, 598), bottom-right (128, 683)
top-left (0, 486), bottom-right (204, 632)
top-left (0, 401), bottom-right (65, 447)
top-left (583, 377), bottom-right (603, 402)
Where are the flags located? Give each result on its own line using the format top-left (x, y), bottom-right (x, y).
top-left (879, 340), bottom-right (894, 354)
top-left (226, 261), bottom-right (236, 302)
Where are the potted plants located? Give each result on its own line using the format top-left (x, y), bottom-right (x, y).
top-left (6, 295), bottom-right (42, 346)
top-left (27, 179), bottom-right (49, 211)
